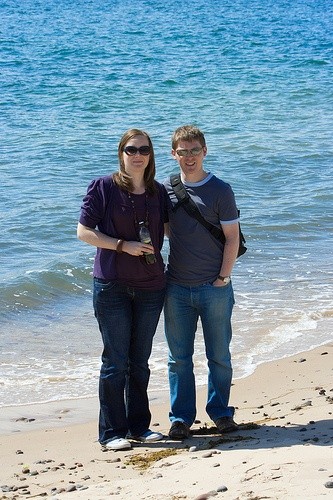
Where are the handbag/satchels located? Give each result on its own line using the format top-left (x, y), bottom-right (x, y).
top-left (237, 209), bottom-right (247, 258)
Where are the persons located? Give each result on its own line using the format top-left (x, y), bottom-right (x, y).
top-left (162, 127), bottom-right (239, 437)
top-left (75, 130), bottom-right (170, 450)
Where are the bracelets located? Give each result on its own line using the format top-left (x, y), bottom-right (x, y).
top-left (117, 239), bottom-right (124, 254)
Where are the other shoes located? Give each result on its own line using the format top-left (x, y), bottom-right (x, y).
top-left (133, 430), bottom-right (163, 441)
top-left (102, 437), bottom-right (131, 452)
top-left (169, 421), bottom-right (190, 439)
top-left (214, 416), bottom-right (238, 433)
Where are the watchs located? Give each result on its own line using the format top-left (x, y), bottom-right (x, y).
top-left (218, 276), bottom-right (231, 284)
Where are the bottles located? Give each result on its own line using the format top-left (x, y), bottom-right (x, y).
top-left (138, 221), bottom-right (157, 265)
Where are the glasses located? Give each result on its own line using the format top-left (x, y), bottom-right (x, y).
top-left (174, 146), bottom-right (204, 157)
top-left (122, 146), bottom-right (151, 156)
